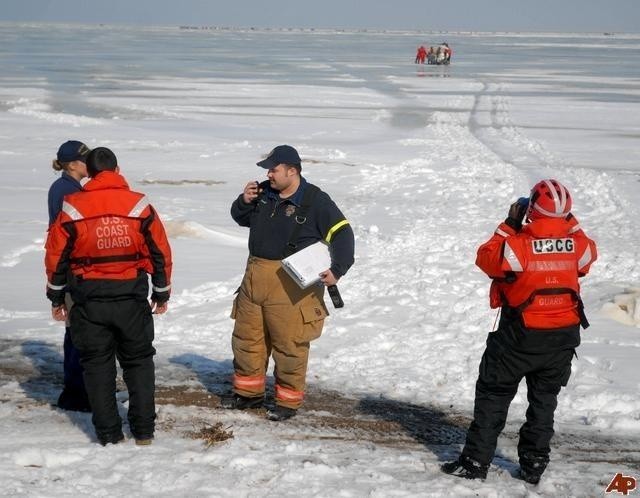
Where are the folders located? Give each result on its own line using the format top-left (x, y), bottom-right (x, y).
top-left (281, 239), bottom-right (336, 291)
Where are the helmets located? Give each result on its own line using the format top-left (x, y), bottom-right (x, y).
top-left (527, 179), bottom-right (572, 223)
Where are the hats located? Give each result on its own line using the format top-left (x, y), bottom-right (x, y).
top-left (256, 145), bottom-right (300, 170)
top-left (57, 141), bottom-right (99, 165)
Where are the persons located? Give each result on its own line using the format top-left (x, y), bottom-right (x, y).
top-left (48, 140), bottom-right (93, 412)
top-left (230, 145), bottom-right (355, 420)
top-left (415, 42), bottom-right (452, 66)
top-left (441, 180), bottom-right (597, 484)
top-left (45, 146), bottom-right (173, 446)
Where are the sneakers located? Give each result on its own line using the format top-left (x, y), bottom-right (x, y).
top-left (265, 405), bottom-right (296, 420)
top-left (131, 433), bottom-right (155, 446)
top-left (441, 457), bottom-right (488, 480)
top-left (517, 461), bottom-right (545, 483)
top-left (102, 434), bottom-right (123, 445)
top-left (221, 393), bottom-right (266, 410)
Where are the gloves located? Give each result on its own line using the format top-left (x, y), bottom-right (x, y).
top-left (505, 197), bottom-right (528, 229)
